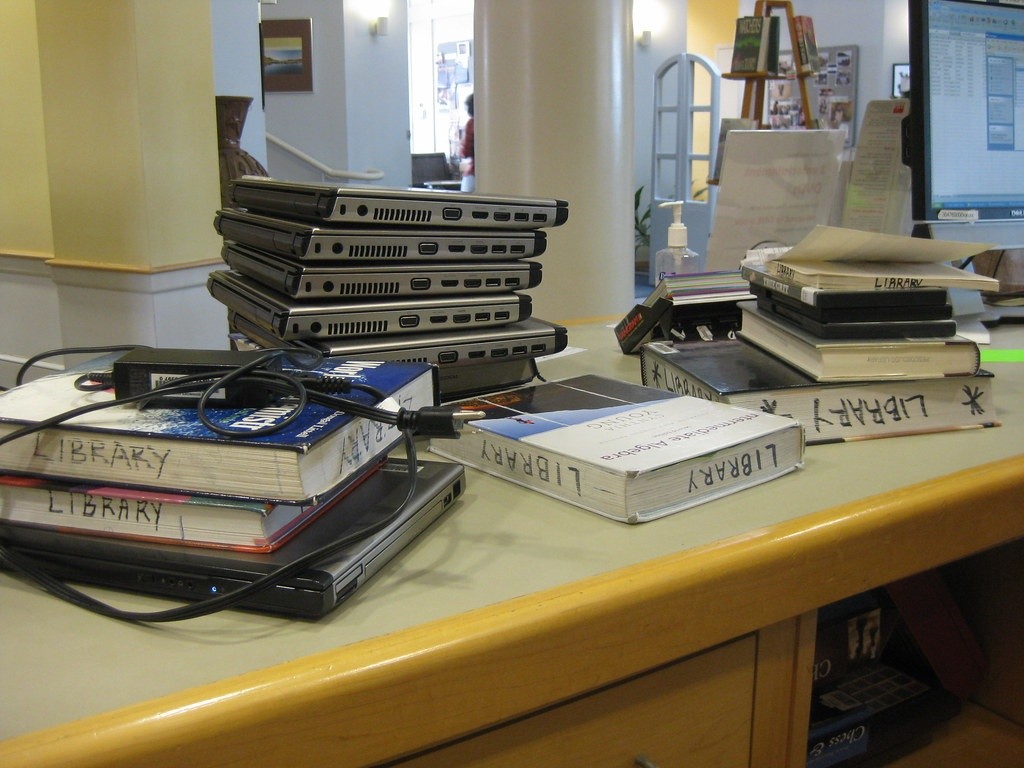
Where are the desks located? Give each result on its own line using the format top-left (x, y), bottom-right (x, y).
top-left (0, 325), bottom-right (1024, 767)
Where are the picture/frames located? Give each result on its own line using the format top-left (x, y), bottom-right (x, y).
top-left (262, 17), bottom-right (315, 91)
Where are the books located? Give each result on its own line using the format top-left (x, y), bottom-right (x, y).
top-left (731, 14), bottom-right (781, 73)
top-left (713, 117), bottom-right (760, 180)
top-left (0, 349), bottom-right (441, 553)
top-left (795, 15), bottom-right (820, 73)
top-left (430, 373), bottom-right (806, 525)
top-left (641, 224), bottom-right (1002, 445)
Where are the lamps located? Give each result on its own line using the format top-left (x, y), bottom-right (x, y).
top-left (370, 15), bottom-right (388, 40)
top-left (637, 30), bottom-right (653, 48)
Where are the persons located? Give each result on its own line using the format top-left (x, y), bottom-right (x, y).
top-left (457, 94), bottom-right (475, 192)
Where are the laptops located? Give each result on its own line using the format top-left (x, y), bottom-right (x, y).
top-left (0, 457), bottom-right (466, 619)
top-left (206, 179), bottom-right (568, 367)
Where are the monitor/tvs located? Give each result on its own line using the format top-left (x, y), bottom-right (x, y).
top-left (410, 153), bottom-right (450, 187)
top-left (907, 0), bottom-right (1024, 307)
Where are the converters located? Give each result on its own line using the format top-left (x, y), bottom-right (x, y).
top-left (113, 348), bottom-right (282, 408)
